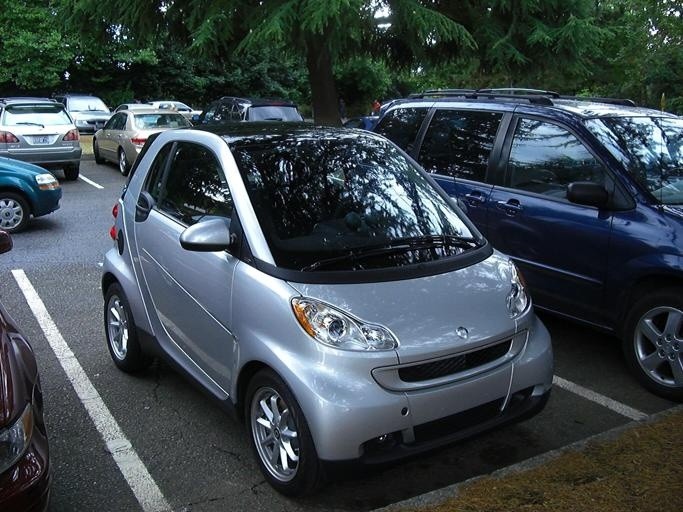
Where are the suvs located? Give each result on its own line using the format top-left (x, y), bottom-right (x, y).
top-left (364, 85), bottom-right (683, 403)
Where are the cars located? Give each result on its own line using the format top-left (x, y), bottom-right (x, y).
top-left (100, 119), bottom-right (556, 496)
top-left (0, 155), bottom-right (63, 233)
top-left (0, 301), bottom-right (51, 511)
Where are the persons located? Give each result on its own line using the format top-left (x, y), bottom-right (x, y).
top-left (338, 97), bottom-right (347, 124)
top-left (371, 99), bottom-right (380, 116)
